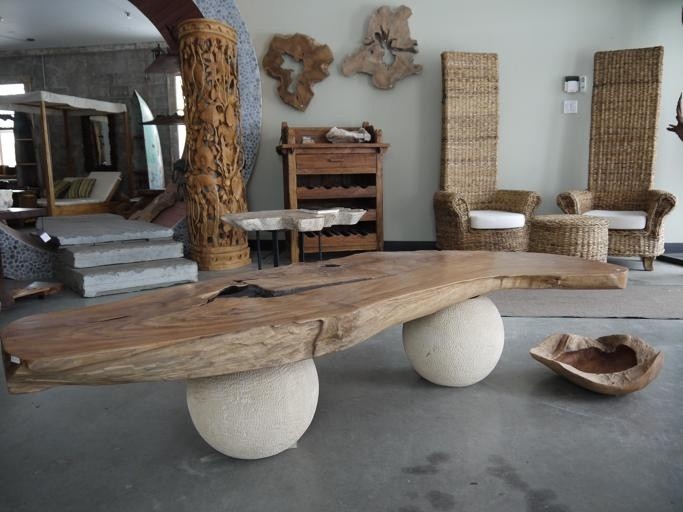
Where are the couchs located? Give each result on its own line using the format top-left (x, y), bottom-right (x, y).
top-left (37, 171), bottom-right (124, 218)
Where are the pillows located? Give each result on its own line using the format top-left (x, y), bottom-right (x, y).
top-left (42, 178), bottom-right (96, 199)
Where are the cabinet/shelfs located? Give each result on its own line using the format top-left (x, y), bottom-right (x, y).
top-left (276, 122), bottom-right (390, 265)
top-left (16, 139), bottom-right (36, 166)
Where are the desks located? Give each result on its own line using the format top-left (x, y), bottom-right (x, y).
top-left (220, 208), bottom-right (366, 270)
top-left (0, 190), bottom-right (24, 207)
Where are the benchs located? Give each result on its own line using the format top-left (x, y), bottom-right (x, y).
top-left (0, 250), bottom-right (629, 459)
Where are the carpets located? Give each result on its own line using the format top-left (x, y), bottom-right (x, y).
top-left (484, 272), bottom-right (683, 318)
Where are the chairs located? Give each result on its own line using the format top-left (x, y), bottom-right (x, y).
top-left (432, 51), bottom-right (541, 252)
top-left (557, 46), bottom-right (677, 271)
